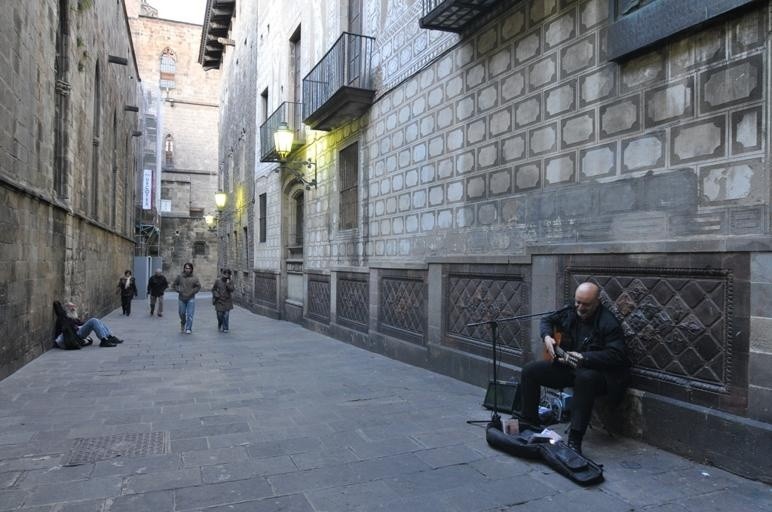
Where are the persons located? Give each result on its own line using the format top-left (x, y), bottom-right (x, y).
top-left (55, 302), bottom-right (122, 349)
top-left (117, 270), bottom-right (137, 316)
top-left (212, 270), bottom-right (234, 333)
top-left (520, 281), bottom-right (627, 455)
top-left (147, 269), bottom-right (167, 317)
top-left (173, 263), bottom-right (201, 334)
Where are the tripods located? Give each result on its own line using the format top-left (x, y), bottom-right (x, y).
top-left (466, 311), bottom-right (555, 423)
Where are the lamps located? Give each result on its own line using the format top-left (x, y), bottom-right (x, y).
top-left (215, 189), bottom-right (241, 226)
top-left (273, 121), bottom-right (317, 191)
top-left (203, 213), bottom-right (220, 233)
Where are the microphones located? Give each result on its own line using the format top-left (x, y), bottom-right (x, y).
top-left (553, 303), bottom-right (572, 316)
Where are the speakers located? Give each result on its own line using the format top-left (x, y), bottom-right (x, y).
top-left (482, 379), bottom-right (522, 413)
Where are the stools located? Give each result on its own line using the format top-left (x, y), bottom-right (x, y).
top-left (563, 387), bottom-right (613, 438)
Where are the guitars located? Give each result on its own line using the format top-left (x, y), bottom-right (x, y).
top-left (544, 326), bottom-right (581, 367)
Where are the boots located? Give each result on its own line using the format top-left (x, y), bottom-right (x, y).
top-left (567, 428), bottom-right (586, 453)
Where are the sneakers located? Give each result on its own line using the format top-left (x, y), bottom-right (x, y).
top-left (100, 338), bottom-right (116, 347)
top-left (108, 336), bottom-right (123, 343)
